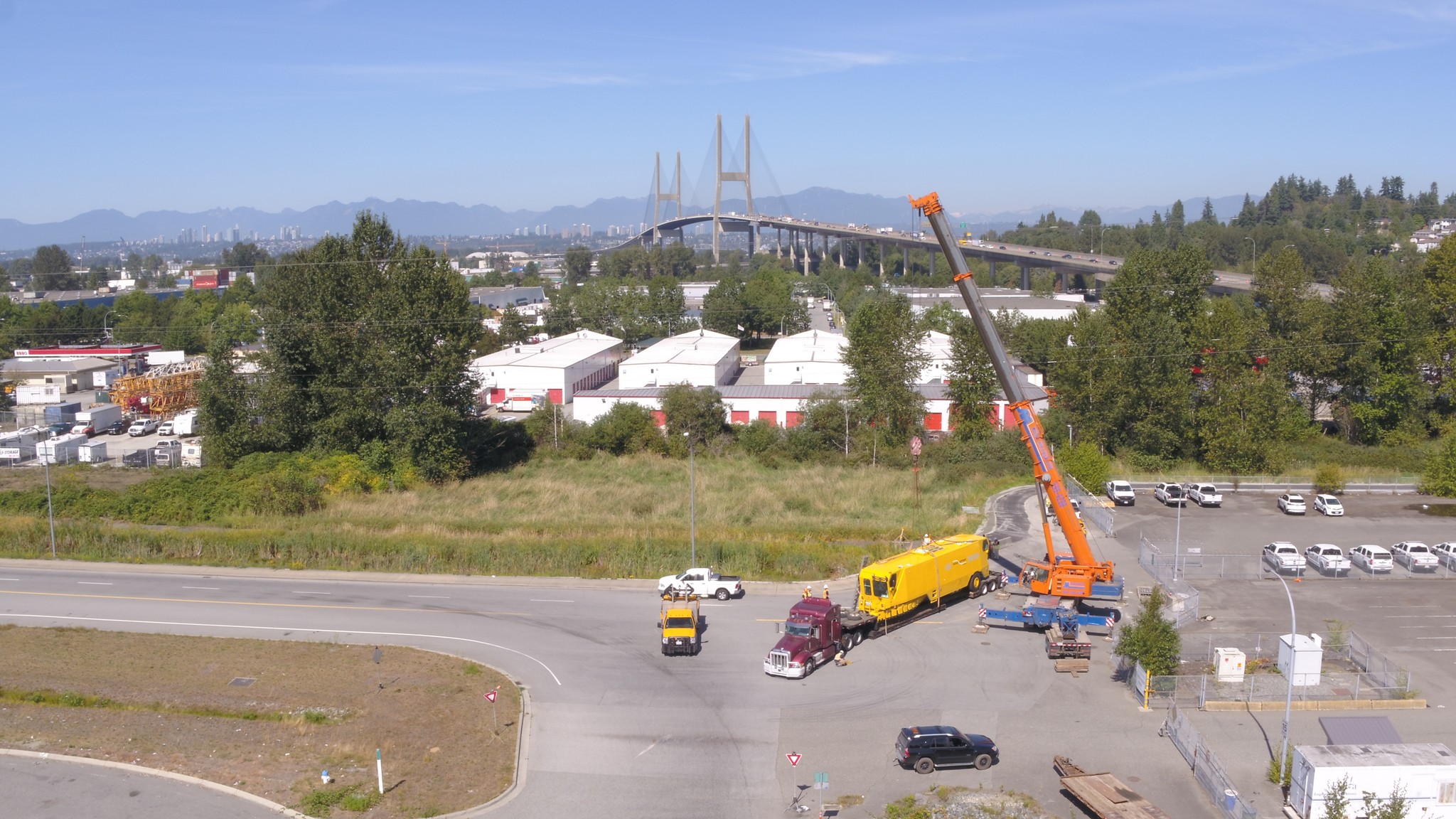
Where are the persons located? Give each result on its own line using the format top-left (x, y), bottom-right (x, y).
top-left (1027, 568), bottom-right (1037, 583)
top-left (993, 538), bottom-right (1000, 560)
top-left (949, 738), bottom-right (955, 747)
top-left (921, 534), bottom-right (931, 546)
top-left (802, 587), bottom-right (808, 601)
top-left (986, 538), bottom-right (992, 559)
top-left (807, 585), bottom-right (812, 597)
top-left (822, 584), bottom-right (829, 599)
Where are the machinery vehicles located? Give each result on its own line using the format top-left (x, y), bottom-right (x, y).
top-left (909, 192), bottom-right (1126, 629)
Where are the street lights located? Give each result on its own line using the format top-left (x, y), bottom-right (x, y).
top-left (820, 283), bottom-right (845, 338)
top-left (104, 311), bottom-right (116, 332)
top-left (839, 400), bottom-right (849, 459)
top-left (1245, 237), bottom-right (1255, 285)
top-left (1084, 224), bottom-right (1110, 264)
top-left (1265, 567), bottom-right (1295, 785)
top-left (18, 426), bottom-right (56, 558)
top-left (1067, 425), bottom-right (1072, 448)
top-left (790, 285), bottom-right (803, 301)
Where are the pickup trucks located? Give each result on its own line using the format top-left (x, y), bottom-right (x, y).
top-left (658, 568), bottom-right (741, 601)
top-left (1105, 480), bottom-right (1223, 506)
top-left (1262, 541), bottom-right (1456, 576)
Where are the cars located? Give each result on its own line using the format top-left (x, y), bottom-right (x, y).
top-left (807, 297), bottom-right (836, 330)
top-left (999, 246), bottom-right (1118, 265)
top-left (719, 212), bottom-right (830, 228)
top-left (4, 384), bottom-right (16, 402)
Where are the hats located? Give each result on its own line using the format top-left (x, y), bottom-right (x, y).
top-left (840, 650), bottom-right (844, 654)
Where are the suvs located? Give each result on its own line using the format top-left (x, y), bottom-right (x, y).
top-left (1068, 498), bottom-right (1081, 519)
top-left (1277, 494), bottom-right (1344, 516)
top-left (657, 583), bottom-right (701, 655)
top-left (895, 726), bottom-right (1000, 774)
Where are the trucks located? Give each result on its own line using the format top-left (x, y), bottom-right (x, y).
top-left (849, 223), bottom-right (927, 240)
top-left (496, 391), bottom-right (550, 413)
top-left (50, 404), bottom-right (207, 468)
top-left (959, 239), bottom-right (994, 249)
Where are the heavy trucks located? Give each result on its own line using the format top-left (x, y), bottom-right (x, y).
top-left (762, 534), bottom-right (1005, 681)
